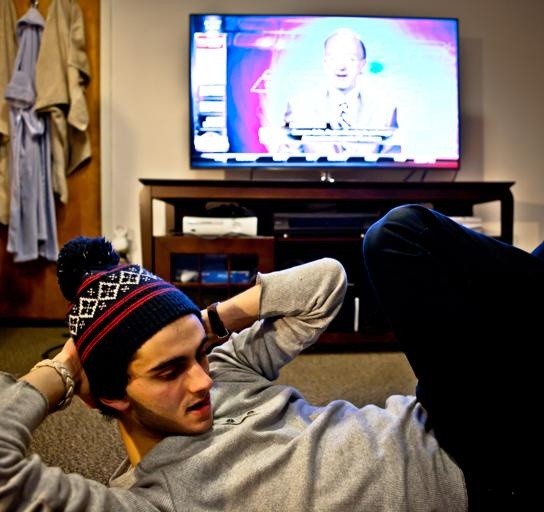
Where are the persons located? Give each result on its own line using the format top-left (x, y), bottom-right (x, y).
top-left (0, 204), bottom-right (544, 511)
top-left (280, 30), bottom-right (401, 155)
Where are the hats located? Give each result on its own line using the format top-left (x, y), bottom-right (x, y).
top-left (56, 235), bottom-right (203, 383)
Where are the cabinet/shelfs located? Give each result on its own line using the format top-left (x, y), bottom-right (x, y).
top-left (139, 177), bottom-right (516, 354)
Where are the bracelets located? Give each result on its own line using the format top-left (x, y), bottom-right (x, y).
top-left (28, 357), bottom-right (74, 412)
top-left (207, 301), bottom-right (231, 343)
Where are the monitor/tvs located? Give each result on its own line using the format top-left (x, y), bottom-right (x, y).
top-left (188, 12), bottom-right (460, 169)
